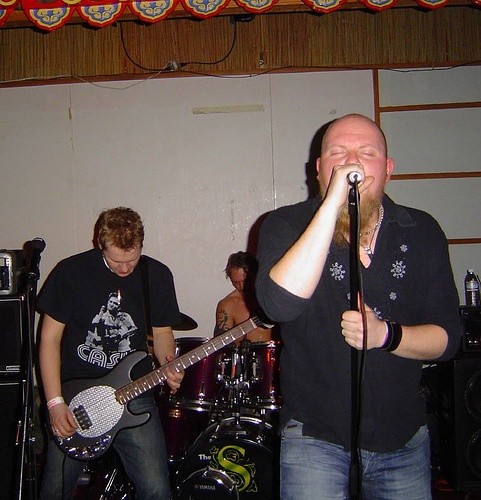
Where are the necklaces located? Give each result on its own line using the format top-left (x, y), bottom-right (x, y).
top-left (360, 206), bottom-right (383, 256)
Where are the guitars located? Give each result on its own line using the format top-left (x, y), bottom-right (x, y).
top-left (44, 316), bottom-right (263, 463)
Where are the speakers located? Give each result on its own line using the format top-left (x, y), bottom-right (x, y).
top-left (0, 292), bottom-right (34, 500)
top-left (419, 354), bottom-right (481, 494)
top-left (457, 306), bottom-right (481, 356)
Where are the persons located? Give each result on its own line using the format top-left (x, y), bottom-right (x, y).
top-left (214, 251), bottom-right (272, 343)
top-left (34, 206), bottom-right (184, 500)
top-left (254, 113), bottom-right (461, 500)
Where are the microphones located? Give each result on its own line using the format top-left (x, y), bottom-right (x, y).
top-left (347, 171), bottom-right (362, 186)
top-left (26, 237), bottom-right (47, 290)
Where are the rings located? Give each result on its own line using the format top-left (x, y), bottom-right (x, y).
top-left (55, 429), bottom-right (59, 432)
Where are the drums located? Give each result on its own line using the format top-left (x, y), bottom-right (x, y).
top-left (169, 335), bottom-right (237, 413)
top-left (245, 339), bottom-right (284, 410)
top-left (183, 417), bottom-right (276, 500)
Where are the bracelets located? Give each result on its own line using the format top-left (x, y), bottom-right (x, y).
top-left (47, 396), bottom-right (65, 409)
top-left (379, 321), bottom-right (402, 351)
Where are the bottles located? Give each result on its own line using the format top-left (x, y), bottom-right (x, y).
top-left (464, 268), bottom-right (481, 307)
